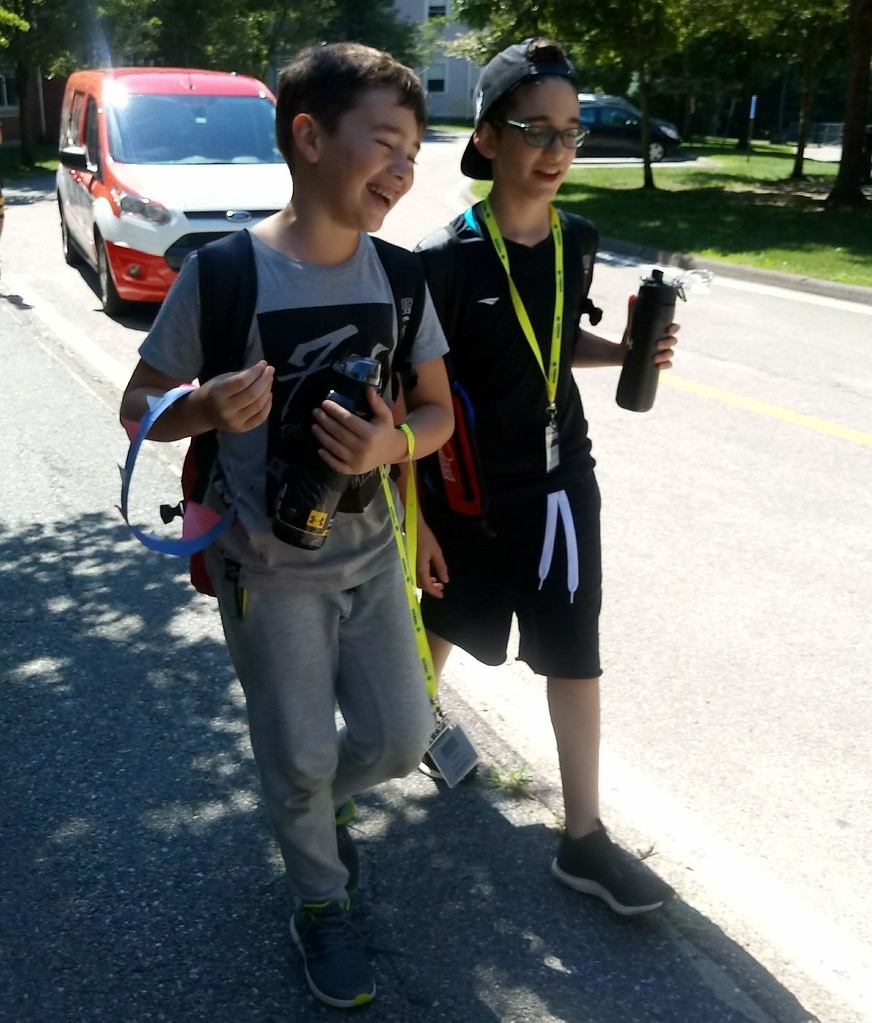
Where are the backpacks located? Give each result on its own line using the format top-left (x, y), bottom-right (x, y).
top-left (161, 229), bottom-right (424, 598)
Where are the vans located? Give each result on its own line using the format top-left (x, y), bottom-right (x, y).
top-left (576, 94), bottom-right (681, 162)
top-left (54, 68), bottom-right (293, 318)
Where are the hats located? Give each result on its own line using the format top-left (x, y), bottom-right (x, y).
top-left (461, 38), bottom-right (578, 181)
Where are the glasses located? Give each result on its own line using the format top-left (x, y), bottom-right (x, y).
top-left (491, 116), bottom-right (590, 149)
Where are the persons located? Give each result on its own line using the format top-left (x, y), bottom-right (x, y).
top-left (122, 44), bottom-right (458, 1007)
top-left (410, 37), bottom-right (678, 913)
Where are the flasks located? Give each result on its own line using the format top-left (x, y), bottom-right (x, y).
top-left (616, 269), bottom-right (686, 412)
top-left (272, 355), bottom-right (384, 551)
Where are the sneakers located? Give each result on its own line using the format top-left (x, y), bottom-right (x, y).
top-left (418, 697), bottom-right (477, 781)
top-left (290, 895), bottom-right (376, 1007)
top-left (551, 815), bottom-right (666, 915)
top-left (332, 797), bottom-right (360, 892)
